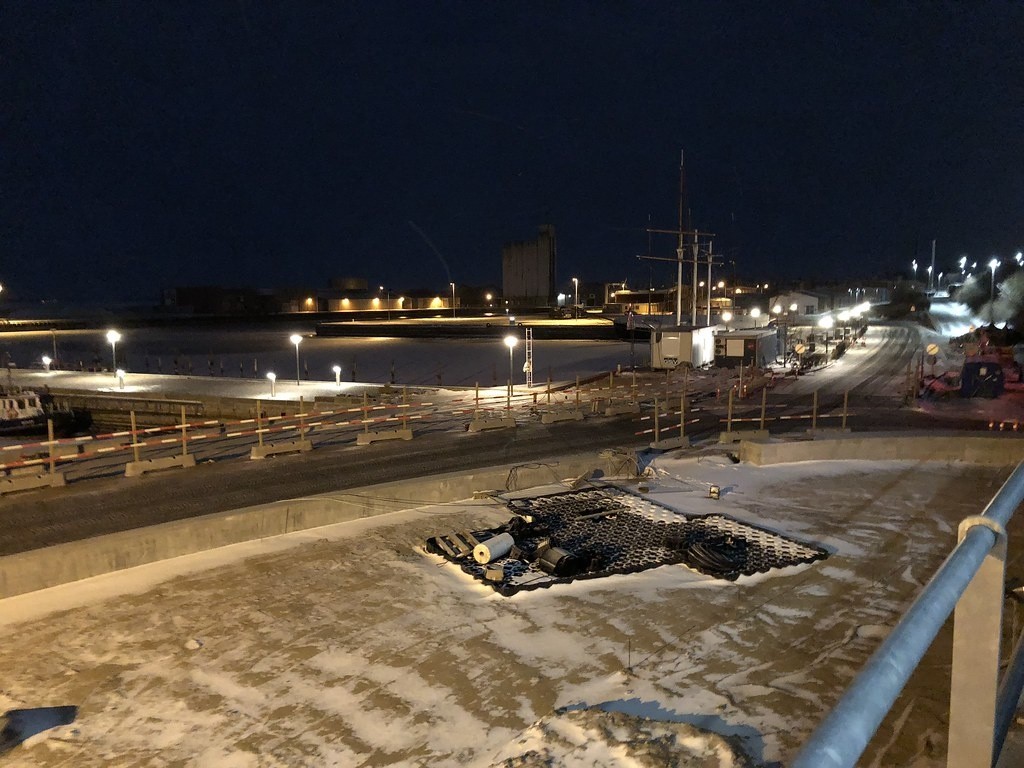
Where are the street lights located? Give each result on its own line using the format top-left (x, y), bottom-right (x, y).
top-left (751, 308), bottom-right (760, 331)
top-left (718, 281), bottom-right (726, 298)
top-left (572, 277), bottom-right (578, 319)
top-left (723, 313), bottom-right (732, 332)
top-left (106, 329), bottom-right (119, 379)
top-left (773, 302), bottom-right (798, 367)
top-left (504, 336), bottom-right (518, 397)
top-left (380, 286), bottom-right (392, 322)
top-left (989, 258), bottom-right (998, 325)
top-left (450, 283), bottom-right (456, 318)
top-left (290, 333), bottom-right (306, 441)
top-left (699, 280), bottom-right (705, 315)
top-left (51, 328), bottom-right (57, 359)
